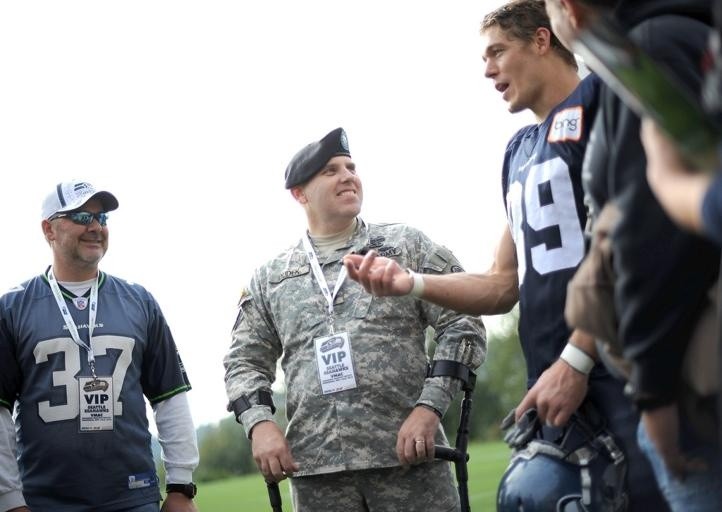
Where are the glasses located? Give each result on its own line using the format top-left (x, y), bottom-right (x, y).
top-left (48, 208), bottom-right (109, 227)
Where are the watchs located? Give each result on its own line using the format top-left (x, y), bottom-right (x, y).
top-left (163, 480), bottom-right (197, 498)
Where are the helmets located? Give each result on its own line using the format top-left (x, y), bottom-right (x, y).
top-left (493, 425), bottom-right (631, 512)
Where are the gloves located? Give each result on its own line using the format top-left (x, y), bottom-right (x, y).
top-left (500, 394), bottom-right (541, 447)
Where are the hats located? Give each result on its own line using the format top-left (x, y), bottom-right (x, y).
top-left (42, 178), bottom-right (120, 221)
top-left (284, 127), bottom-right (350, 190)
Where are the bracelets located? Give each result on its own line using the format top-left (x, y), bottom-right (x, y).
top-left (634, 389), bottom-right (682, 412)
top-left (401, 265), bottom-right (428, 300)
top-left (560, 341), bottom-right (596, 376)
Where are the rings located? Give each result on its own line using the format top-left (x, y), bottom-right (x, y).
top-left (416, 439), bottom-right (425, 443)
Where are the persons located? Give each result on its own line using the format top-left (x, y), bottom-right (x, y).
top-left (531, 0), bottom-right (721, 511)
top-left (637, 112), bottom-right (722, 243)
top-left (223, 125), bottom-right (488, 511)
top-left (342, 0), bottom-right (668, 511)
top-left (1, 181), bottom-right (202, 512)
